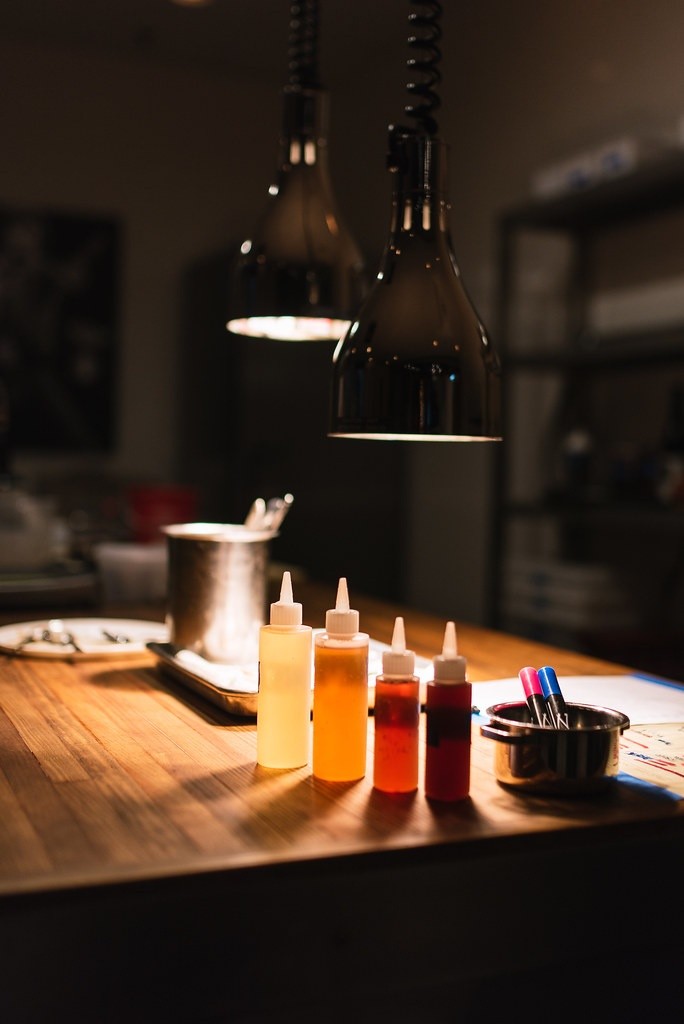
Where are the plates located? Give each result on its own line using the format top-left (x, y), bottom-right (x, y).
top-left (0, 617), bottom-right (171, 660)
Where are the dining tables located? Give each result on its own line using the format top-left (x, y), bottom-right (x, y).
top-left (2, 587), bottom-right (684, 1024)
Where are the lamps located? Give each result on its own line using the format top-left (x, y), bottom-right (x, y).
top-left (225, 0), bottom-right (363, 341)
top-left (327, 0), bottom-right (505, 442)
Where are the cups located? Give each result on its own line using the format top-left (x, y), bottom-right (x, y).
top-left (157, 521), bottom-right (280, 660)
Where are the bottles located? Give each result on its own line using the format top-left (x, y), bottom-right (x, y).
top-left (424, 622), bottom-right (472, 802)
top-left (314, 578), bottom-right (369, 783)
top-left (371, 615), bottom-right (419, 793)
top-left (255, 572), bottom-right (312, 770)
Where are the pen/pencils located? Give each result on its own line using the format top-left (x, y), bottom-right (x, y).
top-left (274, 494), bottom-right (295, 532)
top-left (262, 498), bottom-right (283, 532)
top-left (244, 498), bottom-right (266, 532)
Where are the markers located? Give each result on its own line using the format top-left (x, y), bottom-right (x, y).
top-left (519, 666), bottom-right (553, 727)
top-left (538, 666), bottom-right (574, 729)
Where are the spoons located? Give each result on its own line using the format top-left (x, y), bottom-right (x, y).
top-left (41, 617), bottom-right (86, 655)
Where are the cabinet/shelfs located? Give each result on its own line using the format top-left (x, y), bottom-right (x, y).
top-left (487, 144), bottom-right (684, 676)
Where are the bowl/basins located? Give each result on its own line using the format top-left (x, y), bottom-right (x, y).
top-left (480, 699), bottom-right (631, 798)
